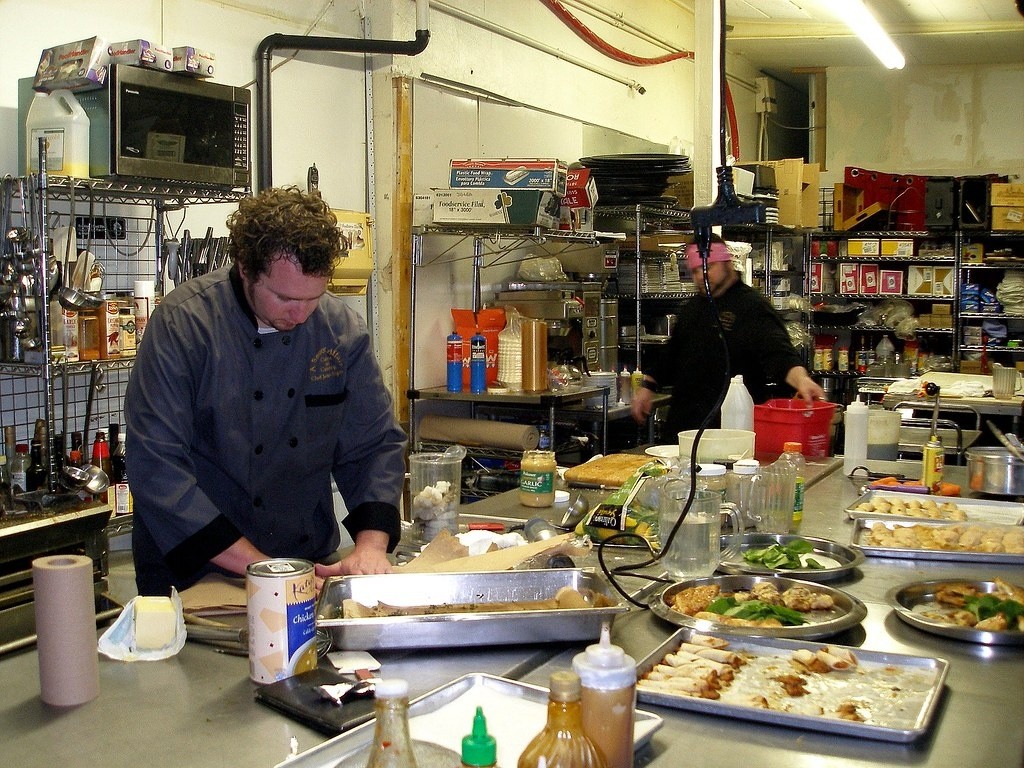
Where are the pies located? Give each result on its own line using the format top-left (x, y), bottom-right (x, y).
top-left (693, 612), bottom-right (781, 627)
top-left (677, 585), bottom-right (720, 615)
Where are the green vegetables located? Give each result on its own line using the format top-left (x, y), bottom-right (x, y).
top-left (741, 539), bottom-right (826, 570)
top-left (577, 498), bottom-right (659, 545)
top-left (706, 596), bottom-right (811, 626)
top-left (962, 594), bottom-right (1024, 633)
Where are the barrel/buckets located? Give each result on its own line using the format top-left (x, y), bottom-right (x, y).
top-left (752, 398), bottom-right (837, 460)
top-left (582, 371), bottom-right (618, 410)
top-left (841, 409), bottom-right (903, 462)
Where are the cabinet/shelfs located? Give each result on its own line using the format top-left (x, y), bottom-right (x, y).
top-left (407, 204), bottom-right (1024, 523)
top-left (0, 137), bottom-right (320, 535)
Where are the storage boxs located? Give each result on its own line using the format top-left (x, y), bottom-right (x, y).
top-left (429, 157), bottom-right (600, 230)
top-left (734, 157), bottom-right (1024, 328)
top-left (30, 35), bottom-right (216, 93)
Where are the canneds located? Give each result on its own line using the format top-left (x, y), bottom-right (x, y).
top-left (520, 450), bottom-right (557, 507)
top-left (246, 559), bottom-right (318, 685)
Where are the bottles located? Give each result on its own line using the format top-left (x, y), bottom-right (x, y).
top-left (446, 331), bottom-right (463, 393)
top-left (812, 332), bottom-right (929, 379)
top-left (693, 464), bottom-right (731, 535)
top-left (775, 442), bottom-right (807, 533)
top-left (569, 620), bottom-right (638, 768)
top-left (535, 425), bottom-right (551, 451)
top-left (0, 418), bottom-right (133, 521)
top-left (619, 366), bottom-right (644, 404)
top-left (48, 279), bottom-right (165, 361)
top-left (453, 705), bottom-right (500, 768)
top-left (726, 459), bottom-right (762, 530)
top-left (364, 679), bottom-right (418, 768)
top-left (517, 671), bottom-right (609, 767)
top-left (470, 331), bottom-right (487, 395)
top-left (921, 435), bottom-right (945, 496)
top-left (522, 516), bottom-right (568, 568)
top-left (720, 375), bottom-right (755, 432)
top-left (408, 452), bottom-right (462, 545)
top-left (842, 395), bottom-right (870, 478)
top-left (24, 87), bottom-right (91, 179)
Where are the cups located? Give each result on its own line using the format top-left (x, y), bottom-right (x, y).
top-left (993, 366), bottom-right (1022, 399)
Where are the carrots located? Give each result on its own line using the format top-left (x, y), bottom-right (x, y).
top-left (870, 477), bottom-right (961, 496)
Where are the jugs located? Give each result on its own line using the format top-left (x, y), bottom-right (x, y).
top-left (658, 490), bottom-right (745, 583)
top-left (745, 461), bottom-right (800, 536)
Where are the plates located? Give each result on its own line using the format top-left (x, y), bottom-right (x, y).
top-left (715, 531), bottom-right (866, 581)
top-left (647, 574), bottom-right (867, 641)
top-left (628, 624), bottom-right (950, 745)
top-left (885, 577), bottom-right (1024, 645)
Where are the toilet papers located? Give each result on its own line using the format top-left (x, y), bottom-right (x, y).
top-left (31, 553), bottom-right (103, 709)
top-left (417, 413), bottom-right (540, 451)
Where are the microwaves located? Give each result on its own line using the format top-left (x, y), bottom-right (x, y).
top-left (15, 60), bottom-right (254, 193)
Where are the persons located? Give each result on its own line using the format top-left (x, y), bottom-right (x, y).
top-left (124, 184), bottom-right (411, 598)
top-left (630, 232), bottom-right (826, 447)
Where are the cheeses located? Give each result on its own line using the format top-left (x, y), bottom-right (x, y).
top-left (133, 596), bottom-right (175, 649)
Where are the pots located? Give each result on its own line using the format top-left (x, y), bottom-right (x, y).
top-left (966, 446), bottom-right (1024, 497)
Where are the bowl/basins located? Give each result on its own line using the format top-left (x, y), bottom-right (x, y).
top-left (495, 334), bottom-right (522, 390)
